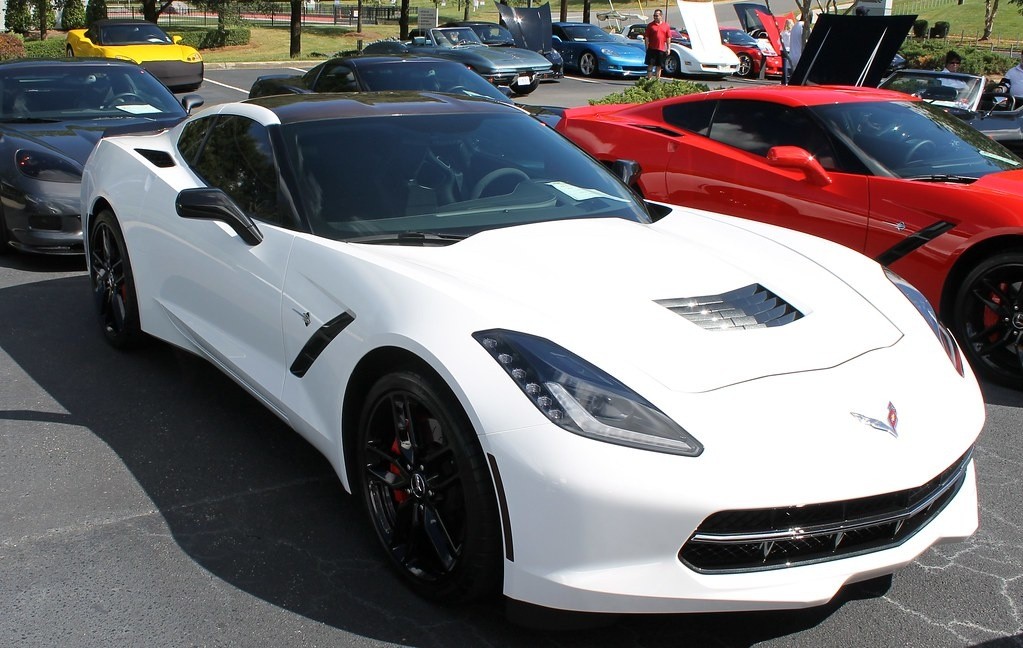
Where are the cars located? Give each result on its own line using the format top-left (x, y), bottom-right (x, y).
top-left (246, 52), bottom-right (571, 132)
top-left (335, 0), bottom-right (1023, 145)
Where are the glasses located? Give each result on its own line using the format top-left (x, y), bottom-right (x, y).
top-left (948, 62), bottom-right (960, 64)
top-left (653, 14), bottom-right (660, 17)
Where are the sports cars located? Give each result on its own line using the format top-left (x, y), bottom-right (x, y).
top-left (78, 89), bottom-right (989, 631)
top-left (549, 86), bottom-right (1023, 393)
top-left (64, 18), bottom-right (204, 92)
top-left (1, 57), bottom-right (206, 264)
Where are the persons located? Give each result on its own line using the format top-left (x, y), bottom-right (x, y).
top-left (643, 8), bottom-right (671, 81)
top-left (936, 50), bottom-right (968, 89)
top-left (992, 50), bottom-right (1023, 97)
top-left (856, 5), bottom-right (871, 16)
top-left (444, 30), bottom-right (460, 45)
top-left (777, 8), bottom-right (814, 85)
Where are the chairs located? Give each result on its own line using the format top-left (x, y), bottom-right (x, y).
top-left (920, 86), bottom-right (959, 100)
top-left (980, 92), bottom-right (1016, 111)
